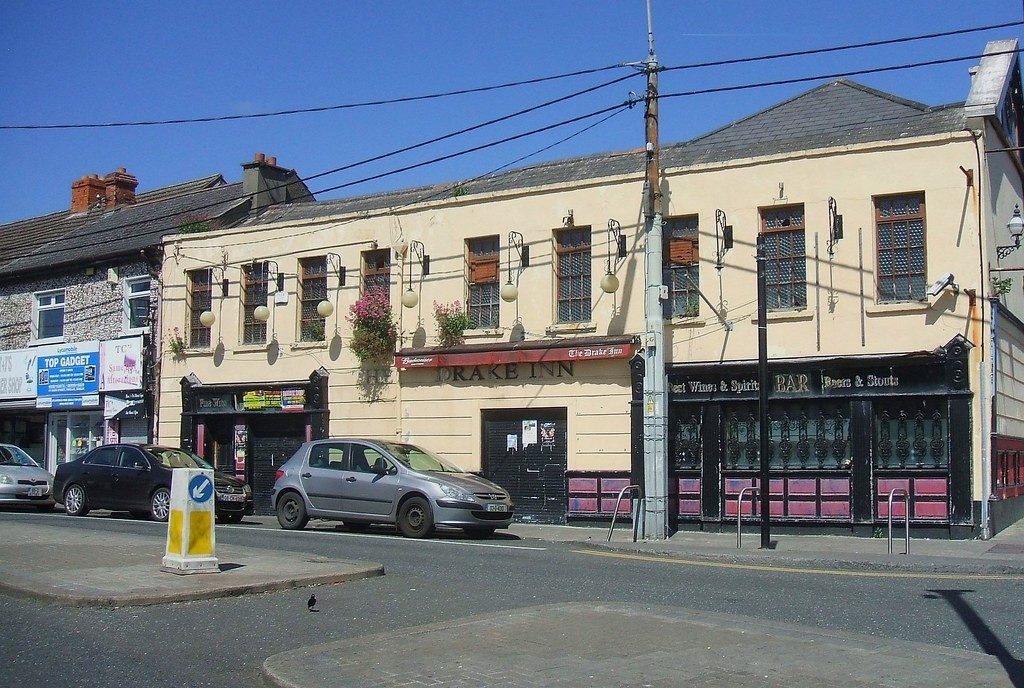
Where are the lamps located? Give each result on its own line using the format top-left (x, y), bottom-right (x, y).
top-left (200, 266), bottom-right (228, 325)
top-left (997, 203), bottom-right (1024, 260)
top-left (317, 252), bottom-right (346, 317)
top-left (501, 230), bottom-right (529, 302)
top-left (402, 241), bottom-right (429, 308)
top-left (84, 266), bottom-right (95, 277)
top-left (254, 260), bottom-right (284, 321)
top-left (601, 218), bottom-right (626, 293)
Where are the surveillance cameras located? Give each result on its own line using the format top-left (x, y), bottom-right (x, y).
top-left (927, 273), bottom-right (954, 296)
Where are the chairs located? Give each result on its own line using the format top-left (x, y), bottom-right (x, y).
top-left (330, 461), bottom-right (342, 468)
top-left (375, 458), bottom-right (387, 468)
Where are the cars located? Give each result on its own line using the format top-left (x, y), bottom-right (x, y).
top-left (270, 438), bottom-right (515, 539)
top-left (53, 442), bottom-right (254, 523)
top-left (0, 444), bottom-right (56, 512)
